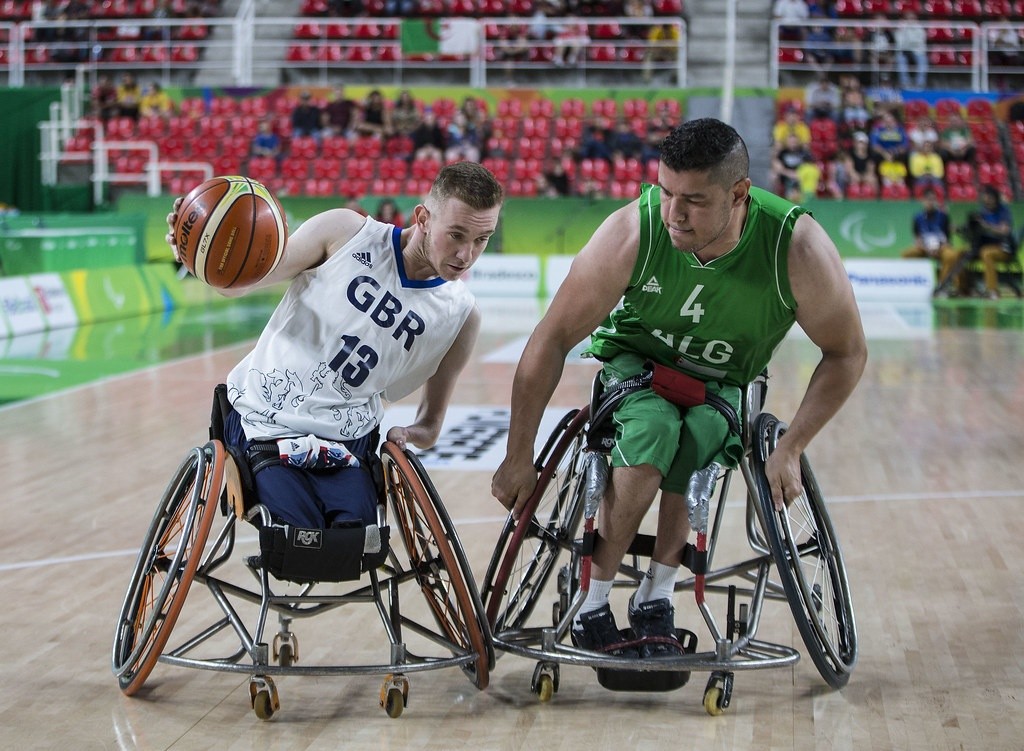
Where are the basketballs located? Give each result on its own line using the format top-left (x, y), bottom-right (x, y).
top-left (172, 172), bottom-right (290, 291)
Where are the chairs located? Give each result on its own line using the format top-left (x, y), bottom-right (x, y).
top-left (0, 1), bottom-right (1024, 201)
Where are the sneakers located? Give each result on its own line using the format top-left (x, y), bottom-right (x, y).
top-left (626, 589), bottom-right (683, 675)
top-left (571, 605), bottom-right (627, 660)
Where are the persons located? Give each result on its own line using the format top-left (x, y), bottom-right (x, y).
top-left (776, 73), bottom-right (980, 200)
top-left (490, 115), bottom-right (868, 673)
top-left (344, 191), bottom-right (407, 229)
top-left (153, 160), bottom-right (504, 538)
top-left (295, 86), bottom-right (495, 169)
top-left (903, 187), bottom-right (954, 297)
top-left (137, 0), bottom-right (182, 45)
top-left (312, 0), bottom-right (420, 17)
top-left (952, 182), bottom-right (1017, 299)
top-left (569, 119), bottom-right (662, 164)
top-left (541, 162), bottom-right (571, 198)
top-left (491, 0), bottom-right (687, 67)
top-left (90, 71), bottom-right (174, 121)
top-left (250, 122), bottom-right (284, 155)
top-left (766, 0), bottom-right (1023, 88)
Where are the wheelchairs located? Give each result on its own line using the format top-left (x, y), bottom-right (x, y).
top-left (462, 357), bottom-right (857, 715)
top-left (111, 382), bottom-right (495, 719)
